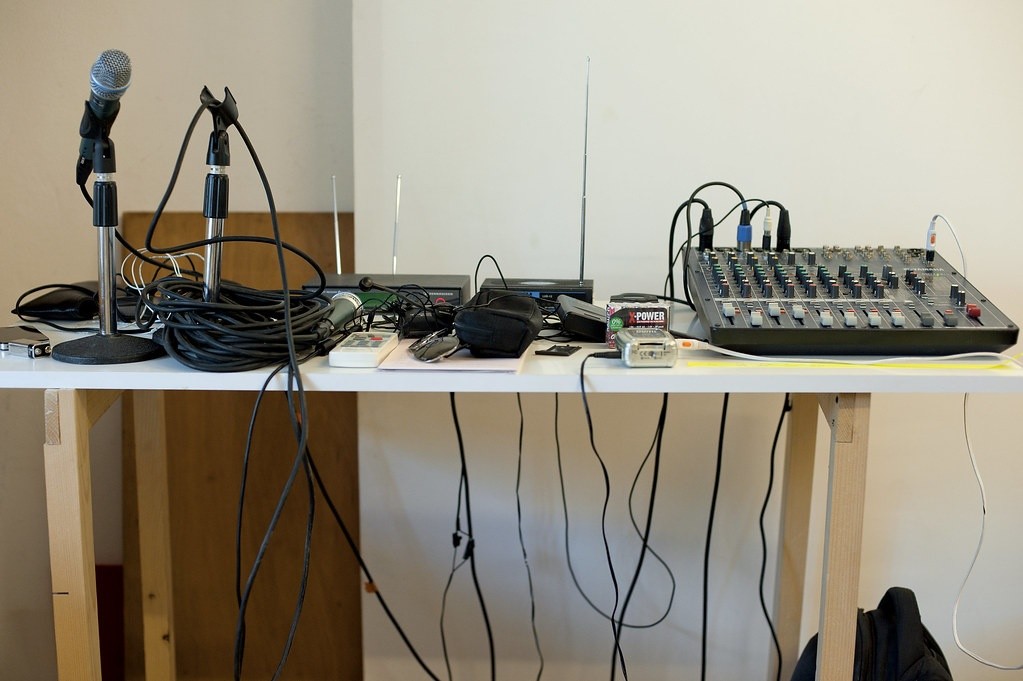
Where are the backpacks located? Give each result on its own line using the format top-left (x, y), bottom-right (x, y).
top-left (791, 586), bottom-right (954, 681)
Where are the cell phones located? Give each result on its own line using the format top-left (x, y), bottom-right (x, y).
top-left (614, 327), bottom-right (677, 367)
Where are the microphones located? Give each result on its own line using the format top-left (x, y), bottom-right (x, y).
top-left (75, 49), bottom-right (132, 185)
top-left (312, 292), bottom-right (363, 341)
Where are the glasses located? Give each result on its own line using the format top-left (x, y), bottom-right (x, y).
top-left (413, 333), bottom-right (471, 363)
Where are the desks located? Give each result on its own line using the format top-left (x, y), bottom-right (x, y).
top-left (0, 313), bottom-right (1023, 681)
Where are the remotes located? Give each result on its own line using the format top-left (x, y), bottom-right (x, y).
top-left (328, 332), bottom-right (399, 367)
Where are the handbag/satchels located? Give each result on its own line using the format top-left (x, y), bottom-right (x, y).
top-left (454, 291), bottom-right (543, 358)
